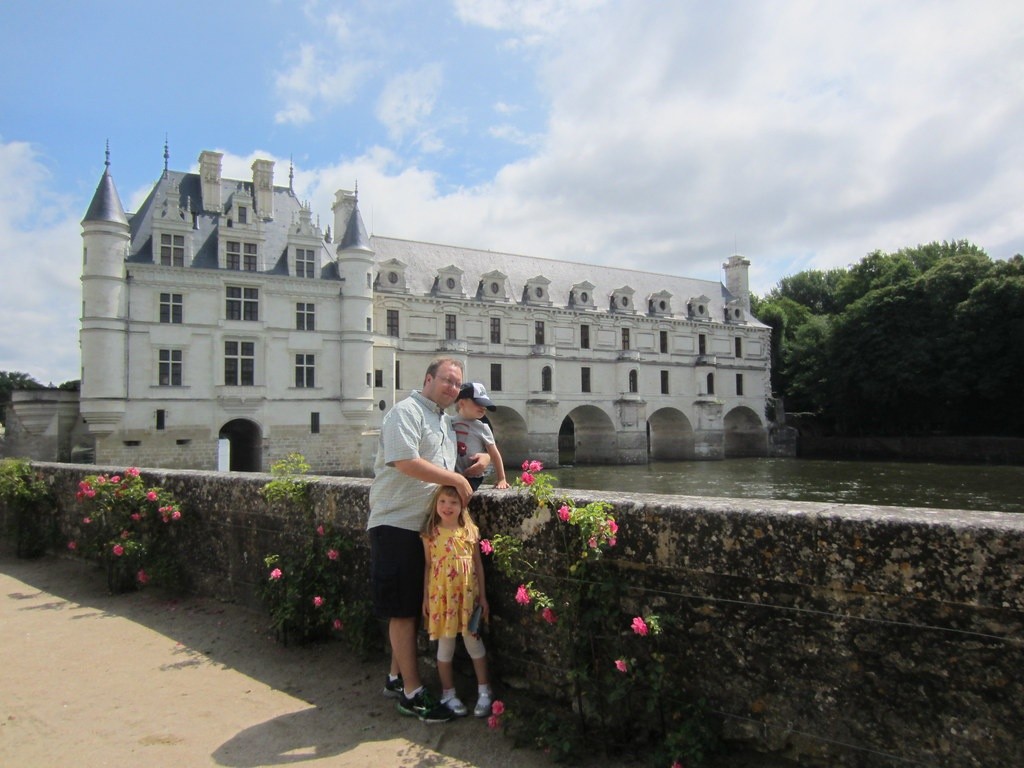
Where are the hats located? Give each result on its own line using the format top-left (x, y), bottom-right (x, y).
top-left (455, 382), bottom-right (497, 412)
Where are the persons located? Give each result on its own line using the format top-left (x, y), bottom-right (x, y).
top-left (366, 358), bottom-right (491, 717)
top-left (451, 383), bottom-right (509, 492)
top-left (420, 484), bottom-right (494, 716)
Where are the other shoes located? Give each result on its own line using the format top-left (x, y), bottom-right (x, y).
top-left (474, 691), bottom-right (497, 717)
top-left (440, 695), bottom-right (466, 716)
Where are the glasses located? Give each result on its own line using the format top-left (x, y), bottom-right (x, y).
top-left (434, 373), bottom-right (464, 391)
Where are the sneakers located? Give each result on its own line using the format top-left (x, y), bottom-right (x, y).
top-left (397, 688), bottom-right (455, 723)
top-left (383, 672), bottom-right (404, 697)
